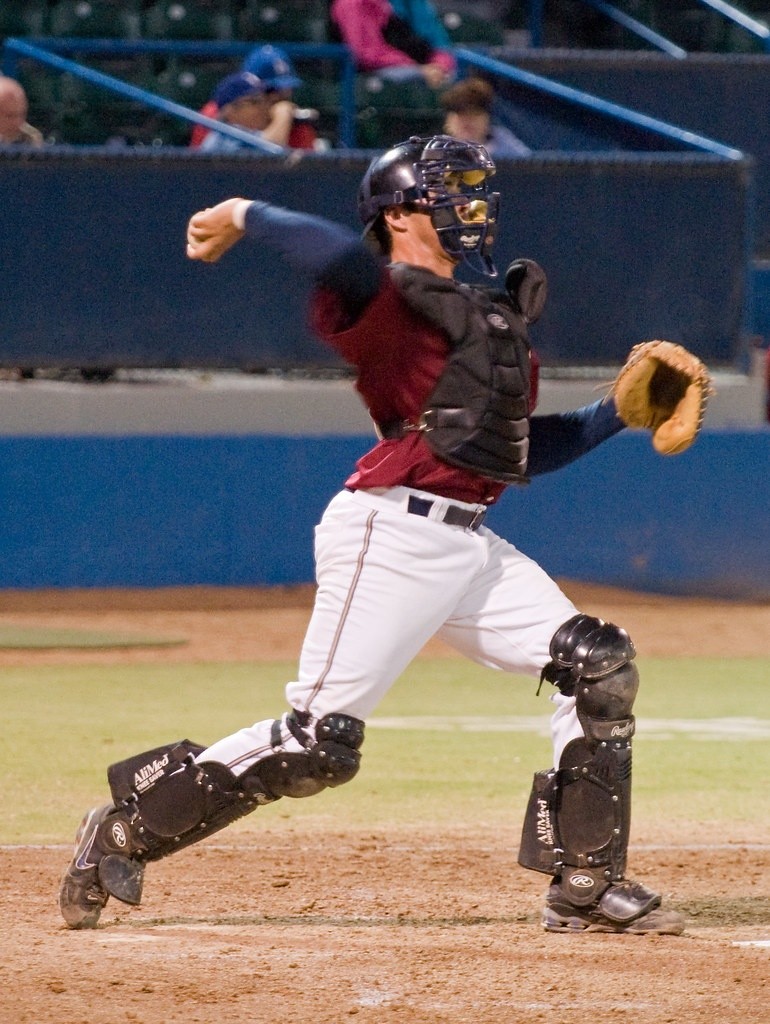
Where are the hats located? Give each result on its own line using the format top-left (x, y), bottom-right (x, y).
top-left (432, 52), bottom-right (458, 74)
top-left (239, 45), bottom-right (306, 89)
top-left (213, 70), bottom-right (279, 108)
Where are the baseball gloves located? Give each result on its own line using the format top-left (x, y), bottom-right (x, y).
top-left (613, 339), bottom-right (710, 456)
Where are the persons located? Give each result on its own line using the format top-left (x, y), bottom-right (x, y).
top-left (436, 76), bottom-right (532, 156)
top-left (0, 75), bottom-right (44, 148)
top-left (327, 0), bottom-right (468, 91)
top-left (191, 43), bottom-right (319, 150)
top-left (57, 134), bottom-right (714, 935)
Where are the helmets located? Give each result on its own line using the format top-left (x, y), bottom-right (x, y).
top-left (358, 137), bottom-right (499, 279)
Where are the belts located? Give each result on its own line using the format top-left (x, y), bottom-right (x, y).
top-left (347, 483), bottom-right (487, 531)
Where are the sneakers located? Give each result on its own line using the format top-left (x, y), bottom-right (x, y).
top-left (542, 869), bottom-right (685, 935)
top-left (59, 804), bottom-right (117, 929)
